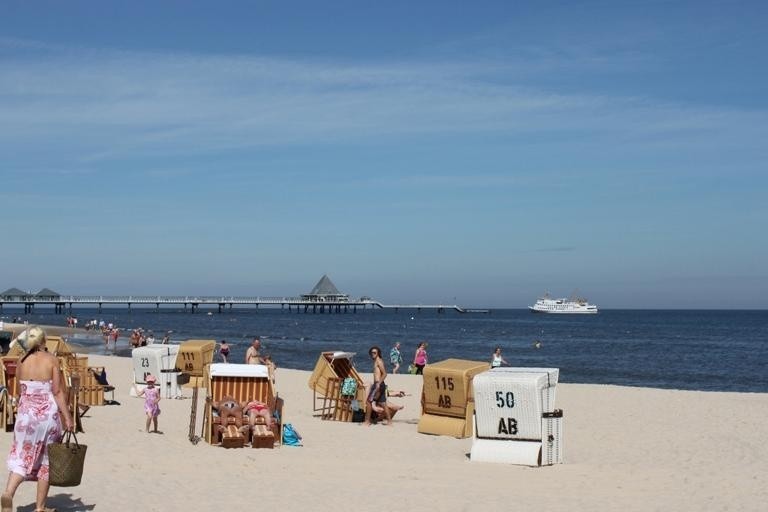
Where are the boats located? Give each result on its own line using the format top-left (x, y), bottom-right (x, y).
top-left (528, 289), bottom-right (599, 314)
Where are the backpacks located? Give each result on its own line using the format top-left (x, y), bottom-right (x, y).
top-left (342, 378), bottom-right (356, 396)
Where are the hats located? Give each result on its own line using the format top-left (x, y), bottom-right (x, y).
top-left (146, 376), bottom-right (156, 382)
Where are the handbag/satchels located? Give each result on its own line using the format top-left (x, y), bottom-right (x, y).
top-left (408, 366), bottom-right (418, 374)
top-left (47, 429), bottom-right (87, 486)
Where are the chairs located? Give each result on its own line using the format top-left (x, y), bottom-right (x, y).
top-left (88, 366), bottom-right (115, 402)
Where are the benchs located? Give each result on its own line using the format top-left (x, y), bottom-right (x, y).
top-left (204, 363), bottom-right (285, 446)
top-left (308, 351), bottom-right (366, 422)
top-left (0, 355), bottom-right (71, 432)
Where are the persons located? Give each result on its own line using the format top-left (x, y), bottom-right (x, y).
top-left (388, 342), bottom-right (403, 374)
top-left (263, 353), bottom-right (276, 388)
top-left (414, 342), bottom-right (427, 374)
top-left (208, 393), bottom-right (249, 433)
top-left (491, 346), bottom-right (512, 369)
top-left (244, 337), bottom-right (264, 365)
top-left (66, 315), bottom-right (169, 351)
top-left (133, 375), bottom-right (162, 433)
top-left (360, 346), bottom-right (392, 425)
top-left (387, 390), bottom-right (406, 397)
top-left (243, 399), bottom-right (275, 432)
top-left (219, 339), bottom-right (231, 363)
top-left (1, 328), bottom-right (74, 511)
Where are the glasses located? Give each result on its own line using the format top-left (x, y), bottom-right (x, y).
top-left (370, 352), bottom-right (376, 354)
